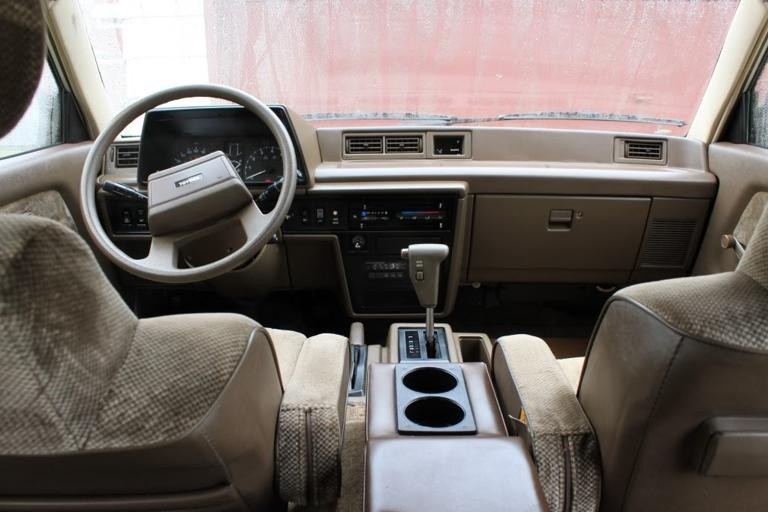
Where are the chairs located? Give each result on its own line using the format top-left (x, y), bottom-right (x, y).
top-left (1, 1), bottom-right (350, 511)
top-left (491, 173), bottom-right (768, 512)
